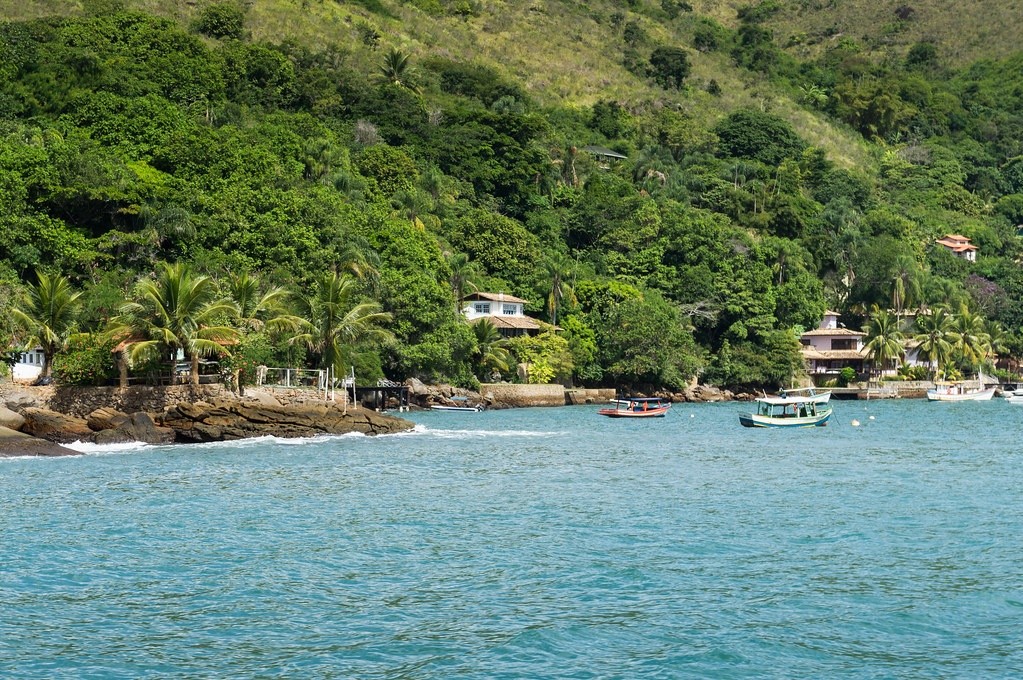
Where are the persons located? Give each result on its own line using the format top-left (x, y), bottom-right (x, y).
top-left (794, 404), bottom-right (801, 417)
top-left (257, 363), bottom-right (267, 385)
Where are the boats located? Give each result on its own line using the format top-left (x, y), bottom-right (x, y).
top-left (926, 383), bottom-right (999, 401)
top-left (598, 396), bottom-right (672, 418)
top-left (430, 396), bottom-right (493, 412)
top-left (738, 389), bottom-right (835, 428)
top-left (994, 385), bottom-right (1023, 406)
